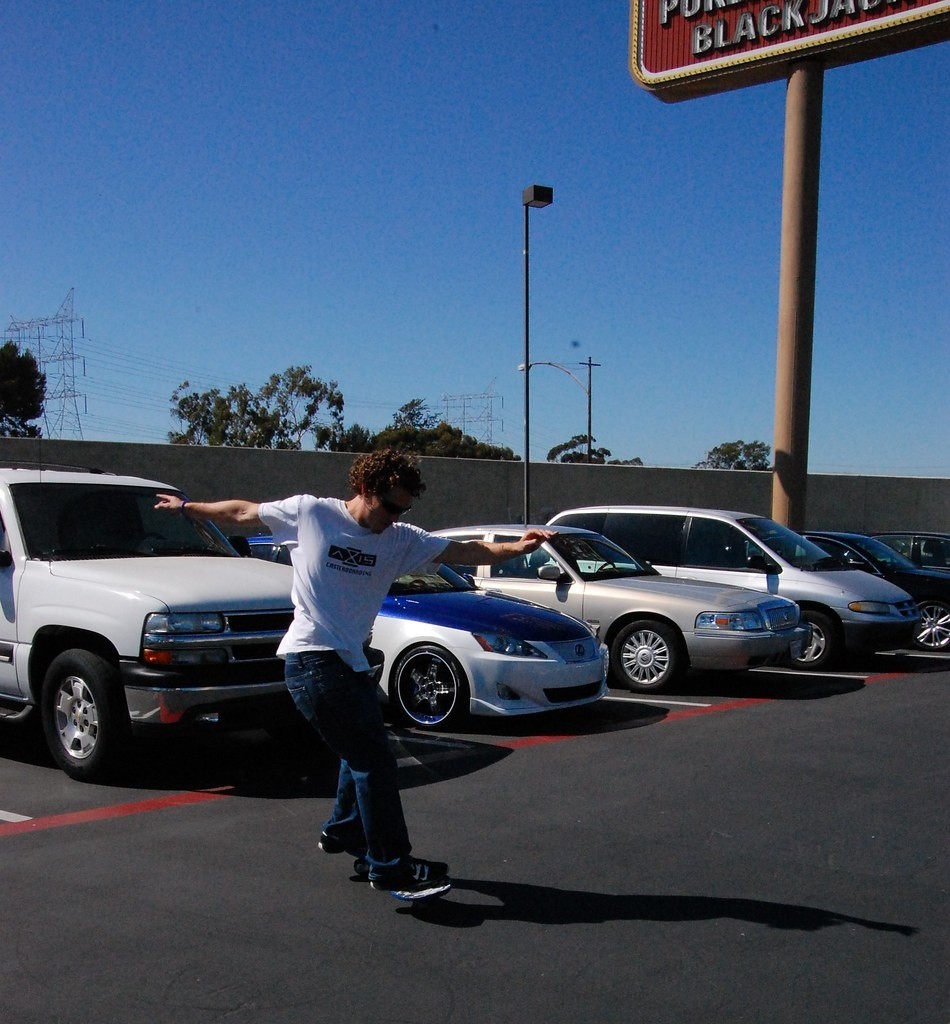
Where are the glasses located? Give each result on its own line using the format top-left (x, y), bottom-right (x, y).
top-left (374, 494), bottom-right (412, 517)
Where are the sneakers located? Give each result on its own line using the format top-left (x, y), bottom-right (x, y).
top-left (370, 855), bottom-right (449, 891)
top-left (317, 827), bottom-right (365, 854)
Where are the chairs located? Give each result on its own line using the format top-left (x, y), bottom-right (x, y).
top-left (921, 540), bottom-right (946, 567)
top-left (528, 550), bottom-right (551, 577)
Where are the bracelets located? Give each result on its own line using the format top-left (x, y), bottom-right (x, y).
top-left (180, 501), bottom-right (189, 514)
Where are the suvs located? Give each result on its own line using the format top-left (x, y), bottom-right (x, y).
top-left (0, 463), bottom-right (385, 782)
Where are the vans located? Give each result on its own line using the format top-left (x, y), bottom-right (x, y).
top-left (524, 505), bottom-right (924, 669)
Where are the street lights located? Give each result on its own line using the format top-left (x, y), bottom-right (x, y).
top-left (518, 356), bottom-right (593, 462)
top-left (523, 183), bottom-right (554, 523)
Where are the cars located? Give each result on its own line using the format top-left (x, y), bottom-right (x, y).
top-left (432, 525), bottom-right (814, 695)
top-left (843, 531), bottom-right (949, 572)
top-left (745, 530), bottom-right (950, 653)
top-left (245, 534), bottom-right (612, 730)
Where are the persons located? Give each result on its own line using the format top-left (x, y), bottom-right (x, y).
top-left (151, 447), bottom-right (551, 894)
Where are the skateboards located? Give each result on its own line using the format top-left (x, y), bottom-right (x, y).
top-left (346, 846), bottom-right (453, 912)
